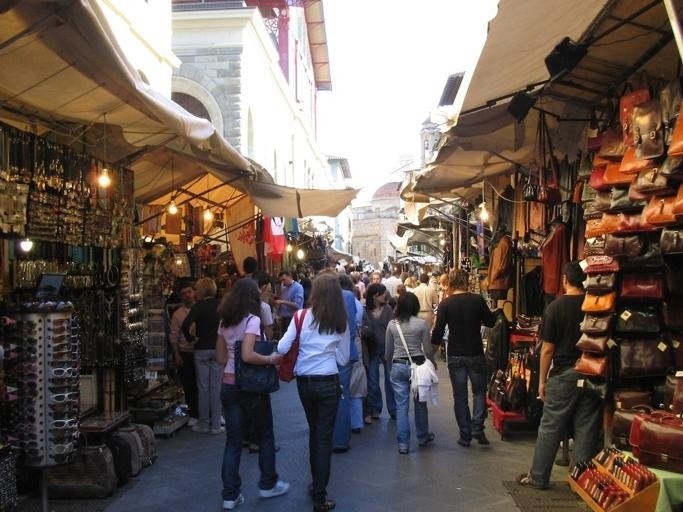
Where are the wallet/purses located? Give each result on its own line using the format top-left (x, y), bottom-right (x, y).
top-left (571, 444), bottom-right (657, 512)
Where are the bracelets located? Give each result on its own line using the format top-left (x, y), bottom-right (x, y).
top-left (266, 355), bottom-right (270, 365)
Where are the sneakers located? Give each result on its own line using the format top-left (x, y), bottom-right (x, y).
top-left (365, 408), bottom-right (380, 424)
top-left (188, 415), bottom-right (226, 435)
top-left (457, 436), bottom-right (470, 446)
top-left (472, 429), bottom-right (490, 445)
top-left (516, 472), bottom-right (548, 490)
top-left (332, 444), bottom-right (351, 454)
top-left (352, 424), bottom-right (363, 433)
top-left (418, 431), bottom-right (436, 446)
top-left (222, 493), bottom-right (245, 510)
top-left (308, 482), bottom-right (336, 512)
top-left (259, 481), bottom-right (290, 498)
top-left (398, 441), bottom-right (409, 454)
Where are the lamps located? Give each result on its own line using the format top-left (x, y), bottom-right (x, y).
top-left (479, 180), bottom-right (489, 221)
top-left (205, 174), bottom-right (212, 220)
top-left (97, 112), bottom-right (112, 187)
top-left (168, 156), bottom-right (178, 215)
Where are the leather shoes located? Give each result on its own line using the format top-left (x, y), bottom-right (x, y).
top-left (243, 438), bottom-right (279, 454)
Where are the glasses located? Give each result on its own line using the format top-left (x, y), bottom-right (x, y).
top-left (48, 316), bottom-right (84, 462)
top-left (2, 315), bottom-right (39, 456)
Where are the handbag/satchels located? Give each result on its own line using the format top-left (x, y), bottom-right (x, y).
top-left (521, 183), bottom-right (561, 204)
top-left (574, 80), bottom-right (682, 411)
top-left (45, 424), bottom-right (158, 498)
top-left (275, 308), bottom-right (307, 382)
top-left (609, 403), bottom-right (683, 474)
top-left (233, 316), bottom-right (281, 395)
top-left (486, 353), bottom-right (527, 412)
top-left (410, 360), bottom-right (438, 394)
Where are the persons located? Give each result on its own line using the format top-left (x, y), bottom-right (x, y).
top-left (242, 257), bottom-right (316, 454)
top-left (214, 278), bottom-right (290, 509)
top-left (275, 273), bottom-right (350, 511)
top-left (516, 260), bottom-right (603, 491)
top-left (321, 255), bottom-right (503, 456)
top-left (165, 276), bottom-right (233, 434)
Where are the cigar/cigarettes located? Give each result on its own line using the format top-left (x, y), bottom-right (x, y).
top-left (536, 396), bottom-right (542, 399)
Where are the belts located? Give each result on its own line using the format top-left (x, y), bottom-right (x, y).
top-left (393, 358), bottom-right (424, 364)
